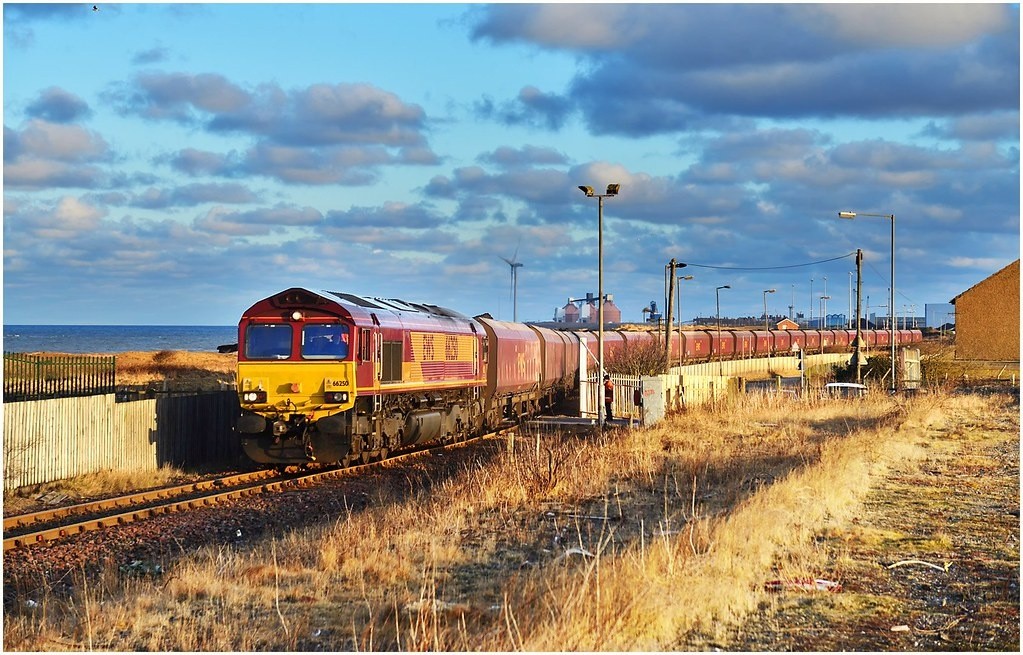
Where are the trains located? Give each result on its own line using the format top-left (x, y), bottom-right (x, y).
top-left (233, 283), bottom-right (924, 472)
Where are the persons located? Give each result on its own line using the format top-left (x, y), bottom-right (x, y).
top-left (604, 374), bottom-right (615, 421)
top-left (319, 332), bottom-right (348, 356)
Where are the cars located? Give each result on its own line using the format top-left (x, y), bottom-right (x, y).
top-left (822, 382), bottom-right (869, 401)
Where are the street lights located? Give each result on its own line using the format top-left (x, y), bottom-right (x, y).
top-left (716, 285), bottom-right (731, 377)
top-left (838, 211), bottom-right (895, 388)
top-left (664, 263), bottom-right (693, 375)
top-left (513, 262), bottom-right (524, 323)
top-left (578, 183), bottom-right (621, 430)
top-left (791, 272), bottom-right (853, 329)
top-left (764, 289), bottom-right (776, 372)
